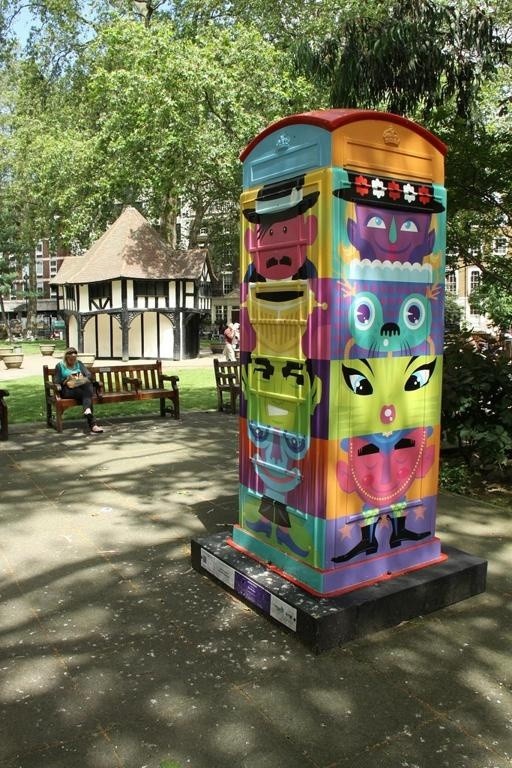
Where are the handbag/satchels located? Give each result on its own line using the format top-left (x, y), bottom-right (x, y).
top-left (67, 376), bottom-right (89, 388)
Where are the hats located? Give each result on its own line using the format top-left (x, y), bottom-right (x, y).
top-left (227, 323), bottom-right (233, 329)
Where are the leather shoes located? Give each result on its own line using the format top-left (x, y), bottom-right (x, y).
top-left (84, 412), bottom-right (92, 417)
top-left (90, 427), bottom-right (103, 433)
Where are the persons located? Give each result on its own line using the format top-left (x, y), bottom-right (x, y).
top-left (222, 322), bottom-right (237, 362)
top-left (53, 347), bottom-right (104, 433)
top-left (233, 319), bottom-right (241, 349)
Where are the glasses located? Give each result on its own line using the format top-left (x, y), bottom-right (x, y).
top-left (67, 351), bottom-right (77, 354)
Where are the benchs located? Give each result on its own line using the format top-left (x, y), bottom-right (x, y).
top-left (213, 358), bottom-right (241, 416)
top-left (43, 359), bottom-right (182, 436)
